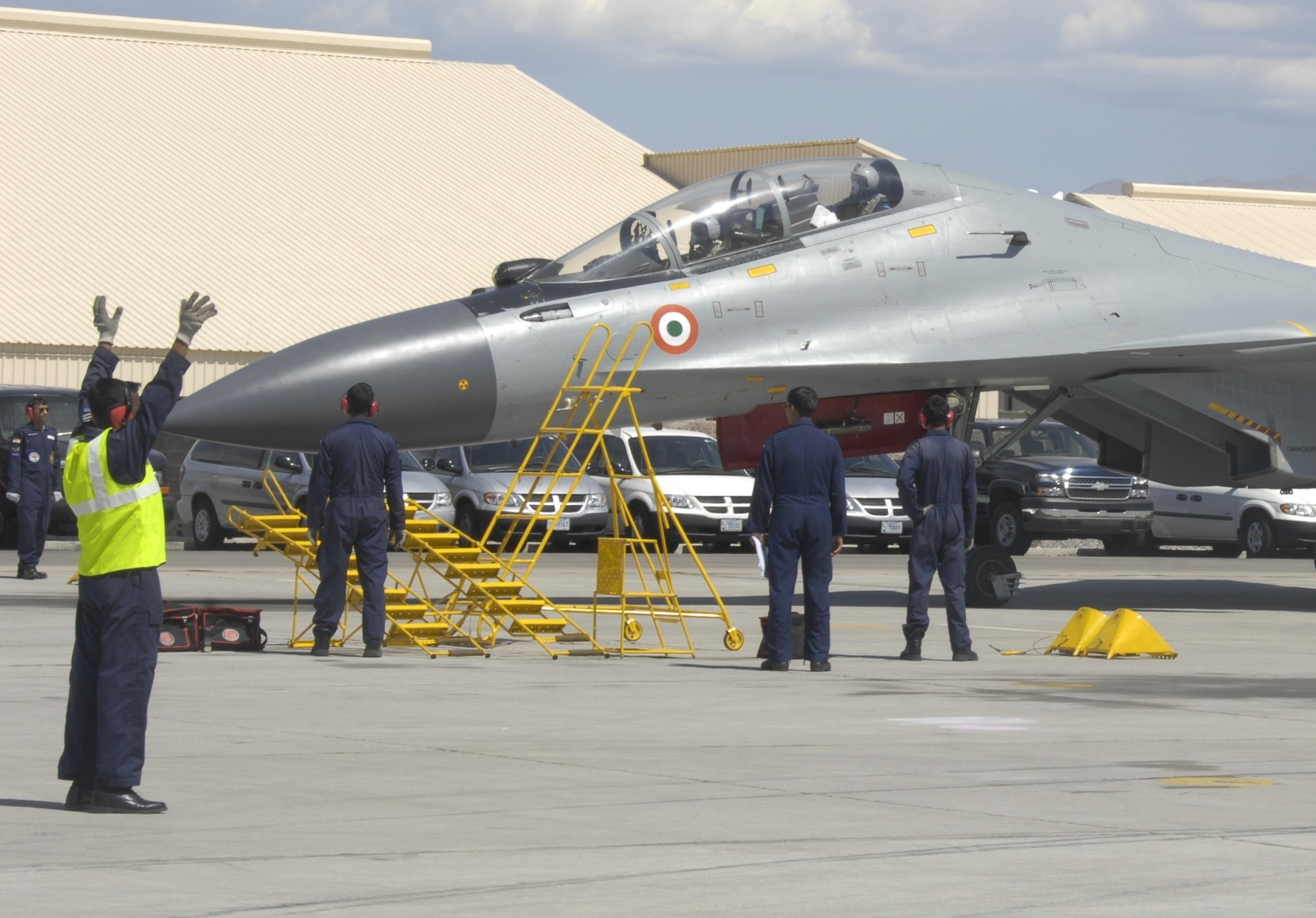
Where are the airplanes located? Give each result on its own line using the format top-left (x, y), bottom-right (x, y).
top-left (152, 154), bottom-right (1316, 607)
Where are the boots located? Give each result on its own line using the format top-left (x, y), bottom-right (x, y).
top-left (900, 638), bottom-right (921, 661)
top-left (310, 634), bottom-right (330, 656)
top-left (363, 643), bottom-right (382, 657)
top-left (952, 649), bottom-right (978, 661)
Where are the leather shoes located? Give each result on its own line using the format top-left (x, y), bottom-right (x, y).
top-left (64, 785), bottom-right (80, 810)
top-left (810, 661), bottom-right (831, 672)
top-left (761, 659), bottom-right (789, 671)
top-left (22, 568), bottom-right (40, 579)
top-left (88, 789), bottom-right (167, 814)
top-left (16, 568), bottom-right (47, 579)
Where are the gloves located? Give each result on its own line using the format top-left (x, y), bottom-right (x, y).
top-left (388, 529), bottom-right (406, 551)
top-left (6, 492), bottom-right (20, 503)
top-left (54, 491), bottom-right (63, 502)
top-left (176, 292), bottom-right (217, 345)
top-left (923, 504), bottom-right (934, 514)
top-left (965, 537), bottom-right (974, 553)
top-left (93, 296), bottom-right (122, 345)
top-left (307, 528), bottom-right (317, 546)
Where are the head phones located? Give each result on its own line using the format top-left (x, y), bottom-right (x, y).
top-left (918, 408), bottom-right (954, 429)
top-left (340, 397), bottom-right (380, 417)
top-left (26, 404), bottom-right (35, 420)
top-left (110, 381), bottom-right (134, 431)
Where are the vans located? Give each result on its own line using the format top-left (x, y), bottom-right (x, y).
top-left (830, 452), bottom-right (913, 552)
top-left (176, 438), bottom-right (457, 551)
top-left (412, 435), bottom-right (610, 552)
top-left (0, 384), bottom-right (174, 549)
top-left (559, 424), bottom-right (771, 554)
top-left (1143, 476), bottom-right (1316, 557)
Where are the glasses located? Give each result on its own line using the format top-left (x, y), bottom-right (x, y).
top-left (38, 408), bottom-right (49, 412)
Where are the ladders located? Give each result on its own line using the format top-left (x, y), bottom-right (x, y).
top-left (378, 494), bottom-right (610, 660)
top-left (435, 321), bottom-right (746, 650)
top-left (228, 468), bottom-right (493, 658)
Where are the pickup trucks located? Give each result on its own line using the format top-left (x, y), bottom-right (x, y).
top-left (944, 414), bottom-right (1156, 557)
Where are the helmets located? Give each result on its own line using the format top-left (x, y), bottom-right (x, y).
top-left (691, 216), bottom-right (721, 244)
top-left (851, 163), bottom-right (879, 192)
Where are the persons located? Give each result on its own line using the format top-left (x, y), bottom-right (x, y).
top-left (891, 393), bottom-right (980, 661)
top-left (6, 397), bottom-right (64, 581)
top-left (743, 385), bottom-right (848, 673)
top-left (53, 290), bottom-right (221, 814)
top-left (818, 162), bottom-right (893, 225)
top-left (684, 215), bottom-right (729, 265)
top-left (305, 382), bottom-right (410, 658)
